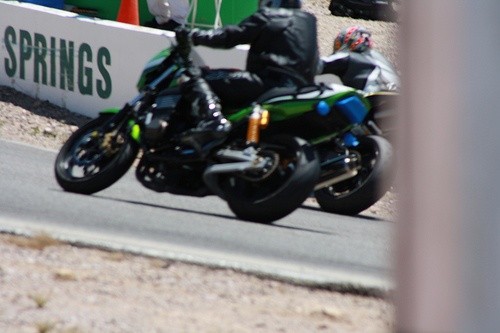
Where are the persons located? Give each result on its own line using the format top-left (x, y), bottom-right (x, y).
top-left (320, 27), bottom-right (397, 91)
top-left (175, 0), bottom-right (318, 132)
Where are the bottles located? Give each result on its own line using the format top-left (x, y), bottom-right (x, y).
top-left (245, 105), bottom-right (262, 144)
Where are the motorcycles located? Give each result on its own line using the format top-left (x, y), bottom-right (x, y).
top-left (52, 25), bottom-right (370, 225)
top-left (312, 88), bottom-right (402, 215)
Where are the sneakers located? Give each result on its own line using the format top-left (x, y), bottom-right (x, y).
top-left (190, 118), bottom-right (232, 133)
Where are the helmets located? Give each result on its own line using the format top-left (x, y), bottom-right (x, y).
top-left (258, 0), bottom-right (301, 8)
top-left (334, 26), bottom-right (373, 53)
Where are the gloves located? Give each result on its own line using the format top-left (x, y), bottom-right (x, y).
top-left (175, 27), bottom-right (198, 46)
